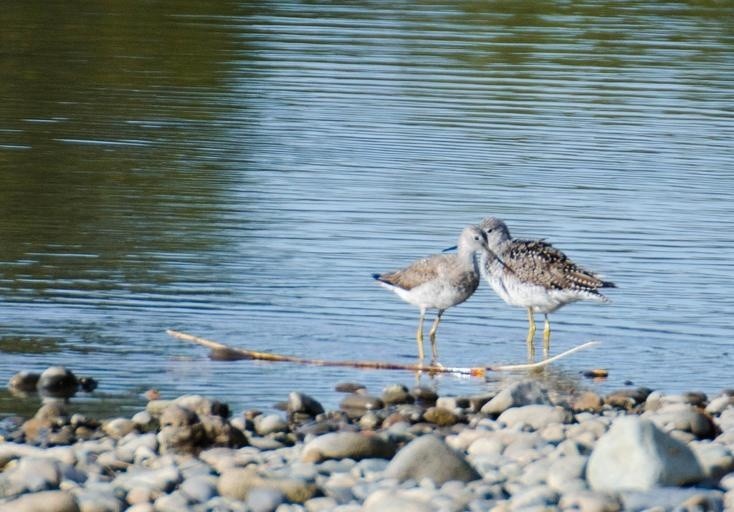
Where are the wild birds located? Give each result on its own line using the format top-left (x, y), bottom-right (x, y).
top-left (440, 215), bottom-right (619, 348)
top-left (370, 223), bottom-right (516, 341)
